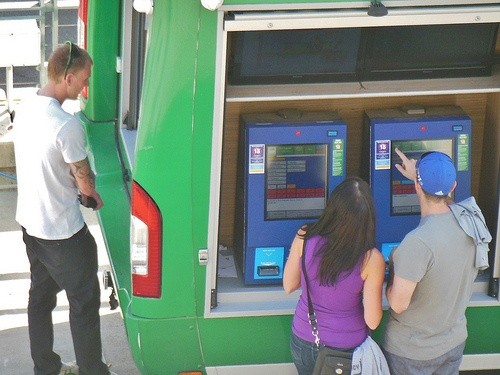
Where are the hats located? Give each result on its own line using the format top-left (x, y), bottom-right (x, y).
top-left (416, 152), bottom-right (457, 196)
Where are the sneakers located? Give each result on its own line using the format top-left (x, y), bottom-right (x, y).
top-left (58, 362), bottom-right (79, 375)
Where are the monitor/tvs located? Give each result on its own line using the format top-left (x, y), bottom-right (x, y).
top-left (391, 138), bottom-right (454, 216)
top-left (266, 144), bottom-right (328, 220)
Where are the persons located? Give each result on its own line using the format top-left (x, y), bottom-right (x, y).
top-left (281, 177), bottom-right (386, 375)
top-left (389, 146), bottom-right (480, 375)
top-left (15, 41), bottom-right (112, 375)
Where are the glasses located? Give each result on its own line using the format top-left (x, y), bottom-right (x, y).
top-left (63, 40), bottom-right (81, 79)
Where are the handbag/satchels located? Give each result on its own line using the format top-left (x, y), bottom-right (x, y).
top-left (313, 347), bottom-right (353, 375)
